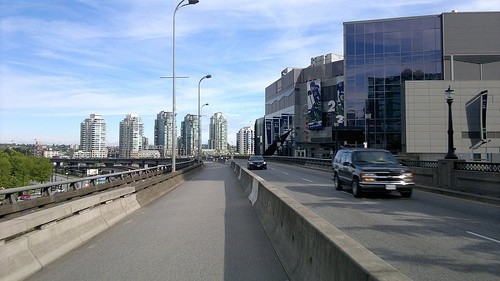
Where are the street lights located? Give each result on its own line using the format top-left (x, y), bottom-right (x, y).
top-left (444, 85), bottom-right (456, 156)
top-left (171, 0), bottom-right (200, 172)
top-left (197, 75), bottom-right (212, 165)
top-left (363, 109), bottom-right (372, 147)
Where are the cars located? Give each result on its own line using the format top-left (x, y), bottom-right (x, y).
top-left (247, 155), bottom-right (269, 169)
top-left (331, 148), bottom-right (416, 196)
